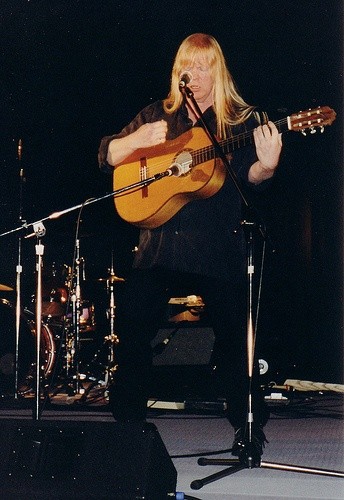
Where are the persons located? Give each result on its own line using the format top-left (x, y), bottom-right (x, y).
top-left (98, 34), bottom-right (282, 456)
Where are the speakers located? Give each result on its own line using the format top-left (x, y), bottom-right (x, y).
top-left (0, 419), bottom-right (177, 500)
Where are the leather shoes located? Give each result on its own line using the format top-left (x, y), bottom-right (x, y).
top-left (231, 422), bottom-right (269, 467)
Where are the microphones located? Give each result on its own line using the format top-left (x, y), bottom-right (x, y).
top-left (154, 162), bottom-right (182, 180)
top-left (178, 71), bottom-right (193, 87)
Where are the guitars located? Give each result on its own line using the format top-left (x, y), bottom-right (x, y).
top-left (113, 106), bottom-right (336, 229)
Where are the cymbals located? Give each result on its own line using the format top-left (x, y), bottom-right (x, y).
top-left (97, 276), bottom-right (126, 282)
top-left (0, 284), bottom-right (13, 291)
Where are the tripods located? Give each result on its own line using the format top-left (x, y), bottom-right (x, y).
top-left (182, 87), bottom-right (344, 491)
top-left (0, 240), bottom-right (125, 403)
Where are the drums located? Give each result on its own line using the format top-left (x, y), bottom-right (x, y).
top-left (62, 298), bottom-right (95, 329)
top-left (32, 288), bottom-right (71, 317)
top-left (0, 307), bottom-right (54, 396)
